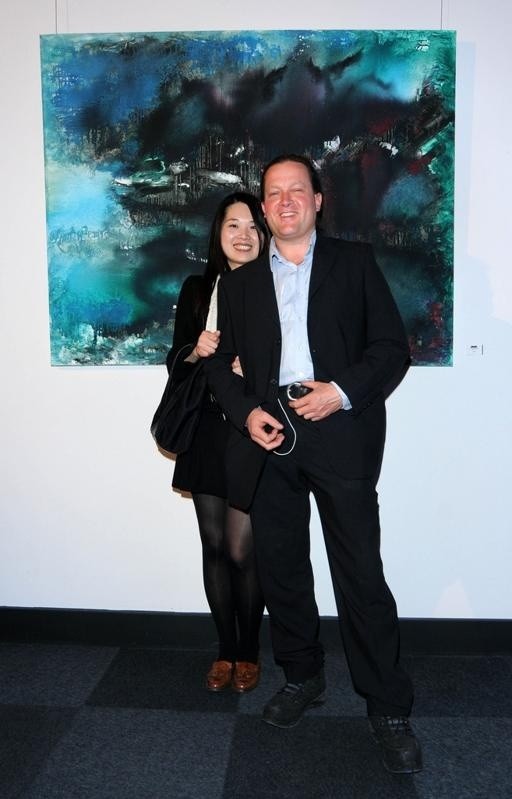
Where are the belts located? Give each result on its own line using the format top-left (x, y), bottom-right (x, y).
top-left (280, 382), bottom-right (312, 403)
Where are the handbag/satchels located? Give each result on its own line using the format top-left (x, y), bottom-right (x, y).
top-left (149, 366), bottom-right (208, 457)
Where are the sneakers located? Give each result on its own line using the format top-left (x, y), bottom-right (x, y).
top-left (372, 715), bottom-right (422, 774)
top-left (263, 669), bottom-right (328, 729)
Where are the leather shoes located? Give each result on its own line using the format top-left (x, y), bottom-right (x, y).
top-left (206, 659), bottom-right (233, 691)
top-left (234, 661), bottom-right (258, 693)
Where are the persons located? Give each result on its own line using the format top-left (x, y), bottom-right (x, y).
top-left (198, 152), bottom-right (426, 779)
top-left (165, 191), bottom-right (275, 700)
